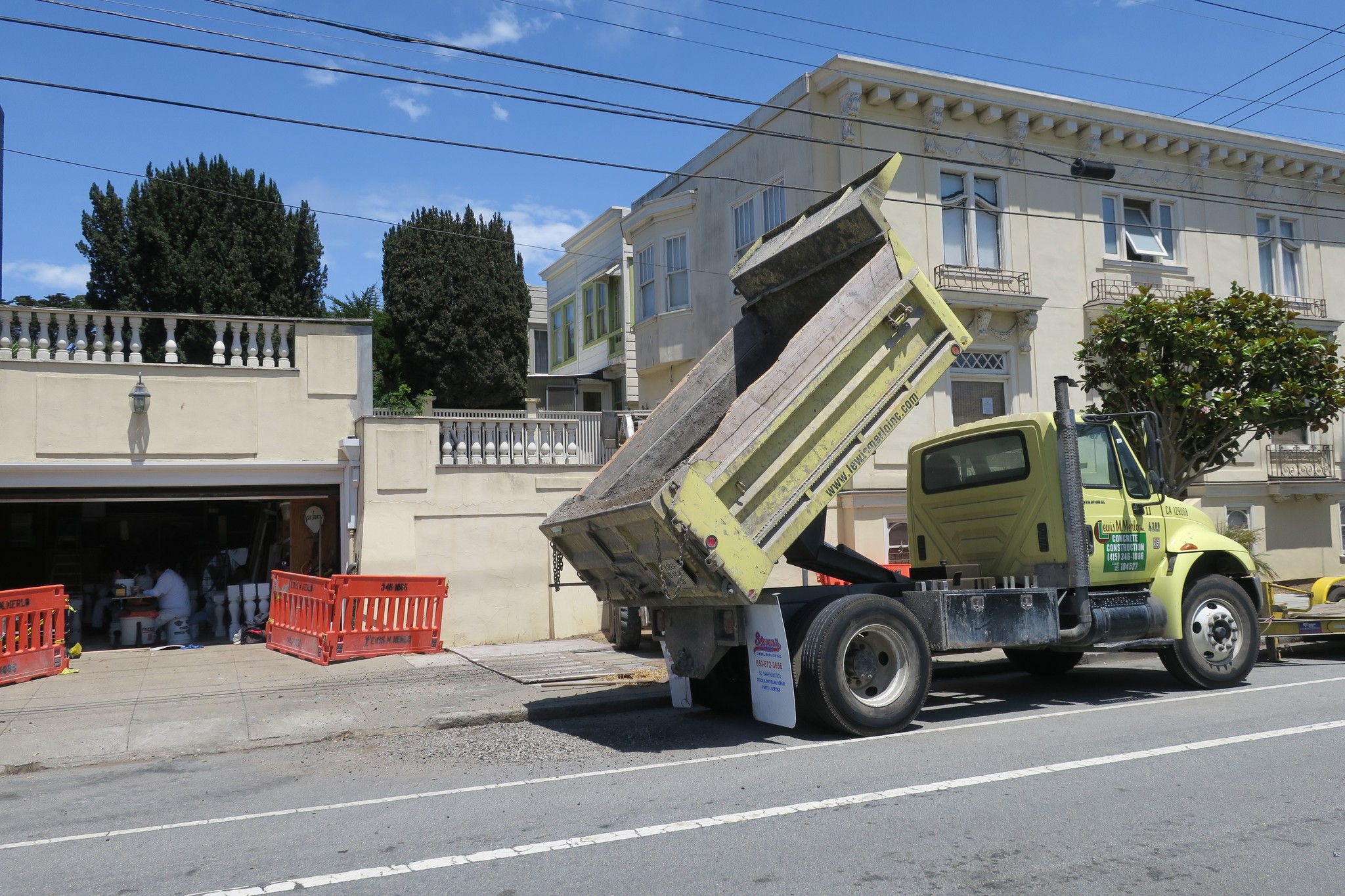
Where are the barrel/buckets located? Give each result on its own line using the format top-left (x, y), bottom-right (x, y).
top-left (115, 579), bottom-right (135, 596)
top-left (119, 616), bottom-right (156, 646)
top-left (167, 616), bottom-right (192, 645)
top-left (136, 576), bottom-right (154, 596)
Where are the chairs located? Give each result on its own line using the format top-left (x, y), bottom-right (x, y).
top-left (924, 453), bottom-right (962, 488)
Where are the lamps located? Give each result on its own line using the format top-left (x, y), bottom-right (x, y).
top-left (128, 372), bottom-right (152, 413)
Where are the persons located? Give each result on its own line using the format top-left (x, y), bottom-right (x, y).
top-left (138, 560), bottom-right (191, 631)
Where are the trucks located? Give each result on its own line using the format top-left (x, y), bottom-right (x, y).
top-left (1260, 578), bottom-right (1345, 663)
top-left (539, 153), bottom-right (1271, 737)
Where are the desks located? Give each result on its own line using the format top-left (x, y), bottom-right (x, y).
top-left (112, 596), bottom-right (152, 609)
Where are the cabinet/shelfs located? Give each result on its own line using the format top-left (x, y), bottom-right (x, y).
top-left (282, 498), bottom-right (337, 574)
